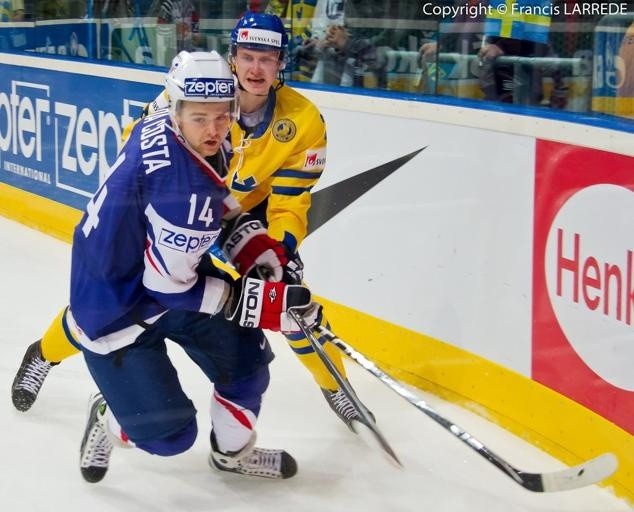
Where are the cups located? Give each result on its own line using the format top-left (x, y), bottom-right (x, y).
top-left (361, 72), bottom-right (376, 89)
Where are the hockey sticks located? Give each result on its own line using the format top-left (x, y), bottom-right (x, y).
top-left (291, 309), bottom-right (401, 470)
top-left (315, 323), bottom-right (616, 492)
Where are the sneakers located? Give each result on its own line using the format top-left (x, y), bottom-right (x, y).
top-left (320, 379), bottom-right (376, 434)
top-left (11, 340), bottom-right (62, 412)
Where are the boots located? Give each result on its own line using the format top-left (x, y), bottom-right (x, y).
top-left (207, 428), bottom-right (297, 478)
top-left (80, 390), bottom-right (132, 484)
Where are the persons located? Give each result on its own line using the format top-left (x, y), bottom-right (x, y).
top-left (2, 1), bottom-right (633, 119)
top-left (12, 13), bottom-right (376, 434)
top-left (67, 49), bottom-right (322, 483)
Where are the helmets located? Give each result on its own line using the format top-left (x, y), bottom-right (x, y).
top-left (230, 11), bottom-right (288, 64)
top-left (165, 50), bottom-right (240, 131)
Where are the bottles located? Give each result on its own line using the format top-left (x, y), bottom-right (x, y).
top-left (340, 58), bottom-right (355, 87)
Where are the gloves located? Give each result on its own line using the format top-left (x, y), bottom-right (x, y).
top-left (223, 220), bottom-right (287, 282)
top-left (224, 272), bottom-right (323, 332)
